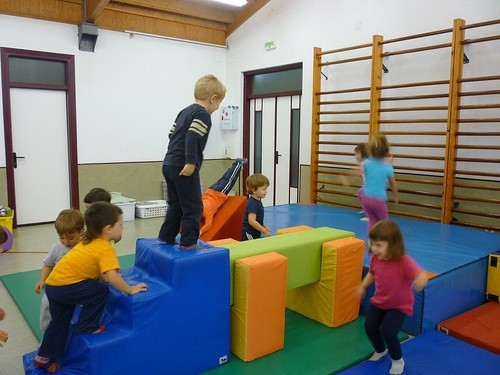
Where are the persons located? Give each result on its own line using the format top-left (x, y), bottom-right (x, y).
top-left (32, 200), bottom-right (147, 375)
top-left (0, 309), bottom-right (8, 347)
top-left (355, 143), bottom-right (369, 221)
top-left (84, 187), bottom-right (114, 246)
top-left (241, 174), bottom-right (271, 239)
top-left (158, 73), bottom-right (226, 248)
top-left (357, 219), bottom-right (428, 375)
top-left (36, 209), bottom-right (83, 349)
top-left (361, 136), bottom-right (398, 233)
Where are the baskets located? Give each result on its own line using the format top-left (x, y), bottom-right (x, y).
top-left (134, 199), bottom-right (169, 219)
